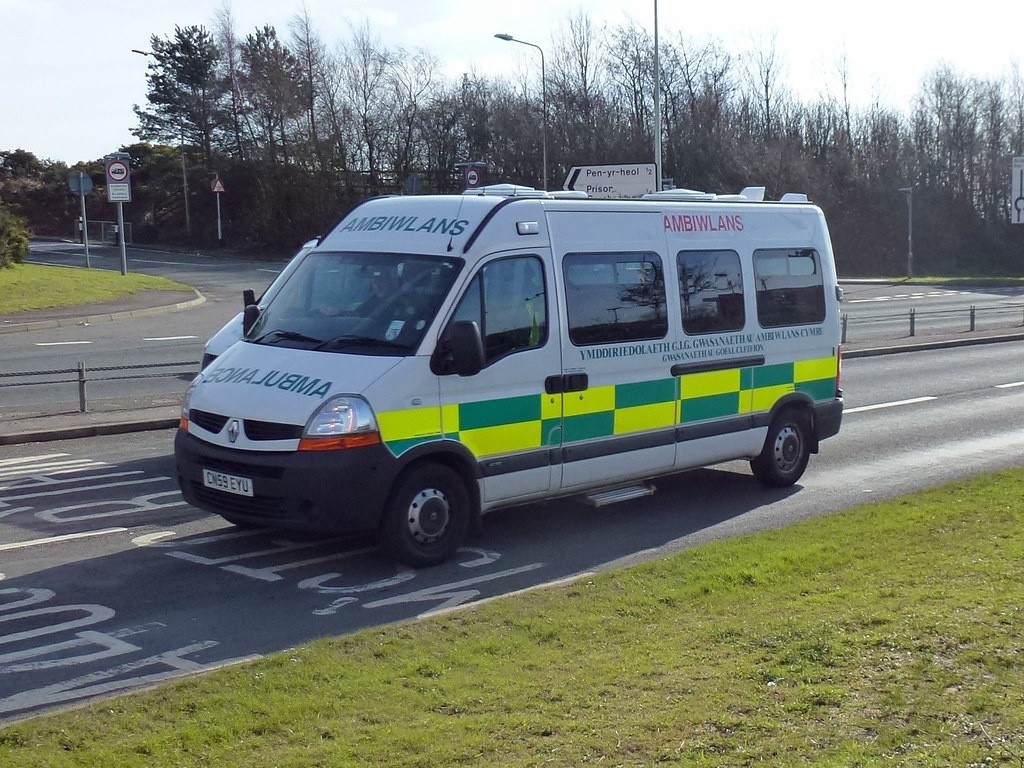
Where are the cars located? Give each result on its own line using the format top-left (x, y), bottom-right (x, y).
top-left (200, 239), bottom-right (318, 375)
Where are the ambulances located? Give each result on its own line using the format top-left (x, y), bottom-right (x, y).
top-left (173, 183), bottom-right (848, 571)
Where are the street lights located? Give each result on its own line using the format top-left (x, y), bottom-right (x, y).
top-left (900, 187), bottom-right (915, 279)
top-left (494, 33), bottom-right (549, 192)
